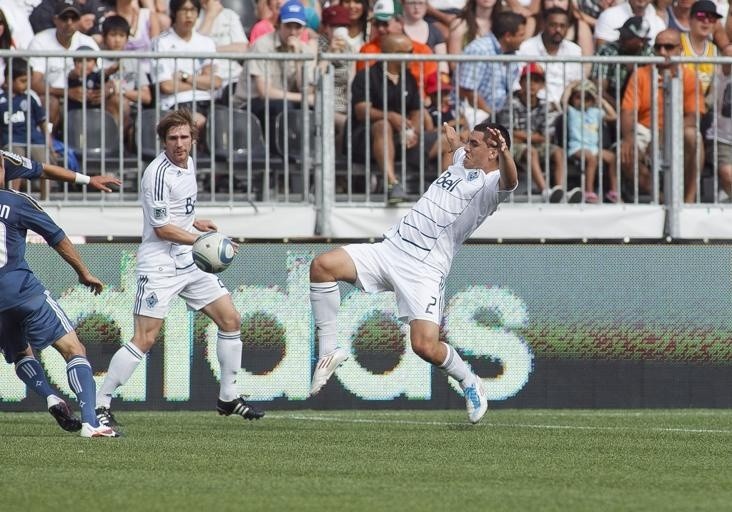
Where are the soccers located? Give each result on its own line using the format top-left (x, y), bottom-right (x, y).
top-left (193, 231), bottom-right (235, 273)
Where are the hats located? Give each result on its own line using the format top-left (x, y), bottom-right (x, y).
top-left (519, 62), bottom-right (544, 83)
top-left (321, 0), bottom-right (405, 25)
top-left (52, 0), bottom-right (81, 19)
top-left (279, 0), bottom-right (307, 26)
top-left (613, 15), bottom-right (653, 41)
top-left (689, 0), bottom-right (724, 19)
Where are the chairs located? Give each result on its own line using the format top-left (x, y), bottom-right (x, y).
top-left (220, 1), bottom-right (259, 38)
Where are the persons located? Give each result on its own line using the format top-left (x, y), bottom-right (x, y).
top-left (94, 111), bottom-right (266, 427)
top-left (1, 0), bottom-right (732, 207)
top-left (0, 152), bottom-right (125, 437)
top-left (308, 120), bottom-right (519, 424)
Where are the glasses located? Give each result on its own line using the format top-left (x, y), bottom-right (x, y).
top-left (55, 14), bottom-right (79, 22)
top-left (652, 43), bottom-right (682, 52)
top-left (692, 11), bottom-right (718, 24)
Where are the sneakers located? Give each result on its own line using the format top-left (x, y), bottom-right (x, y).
top-left (46, 393), bottom-right (82, 433)
top-left (462, 374), bottom-right (489, 425)
top-left (308, 345), bottom-right (351, 398)
top-left (387, 178), bottom-right (405, 206)
top-left (80, 419), bottom-right (121, 438)
top-left (216, 395), bottom-right (266, 421)
top-left (540, 183), bottom-right (628, 203)
top-left (94, 407), bottom-right (123, 427)
